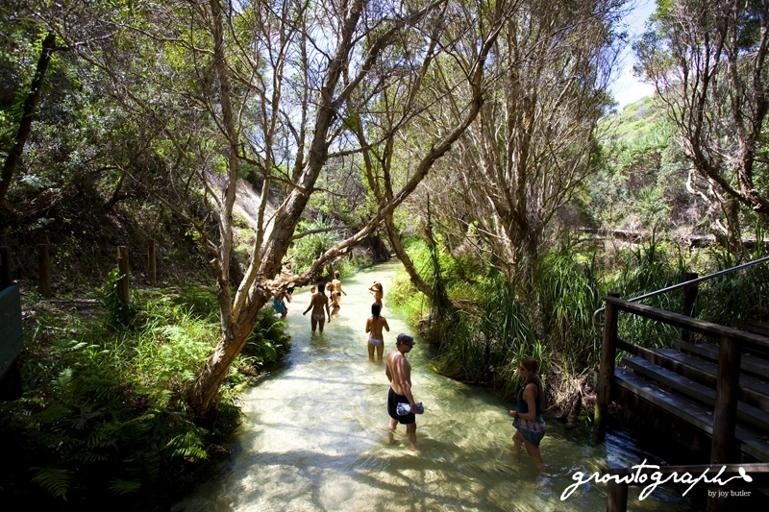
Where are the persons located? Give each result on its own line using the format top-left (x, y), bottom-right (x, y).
top-left (309, 285), bottom-right (315, 295)
top-left (303, 284), bottom-right (332, 337)
top-left (326, 285), bottom-right (341, 315)
top-left (331, 270), bottom-right (348, 303)
top-left (273, 287), bottom-right (292, 319)
top-left (366, 302), bottom-right (390, 365)
top-left (368, 281), bottom-right (385, 312)
top-left (506, 356), bottom-right (546, 474)
top-left (385, 333), bottom-right (424, 443)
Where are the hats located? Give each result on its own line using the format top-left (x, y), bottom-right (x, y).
top-left (397, 333), bottom-right (416, 345)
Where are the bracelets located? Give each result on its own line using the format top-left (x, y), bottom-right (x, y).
top-left (515, 412), bottom-right (518, 418)
